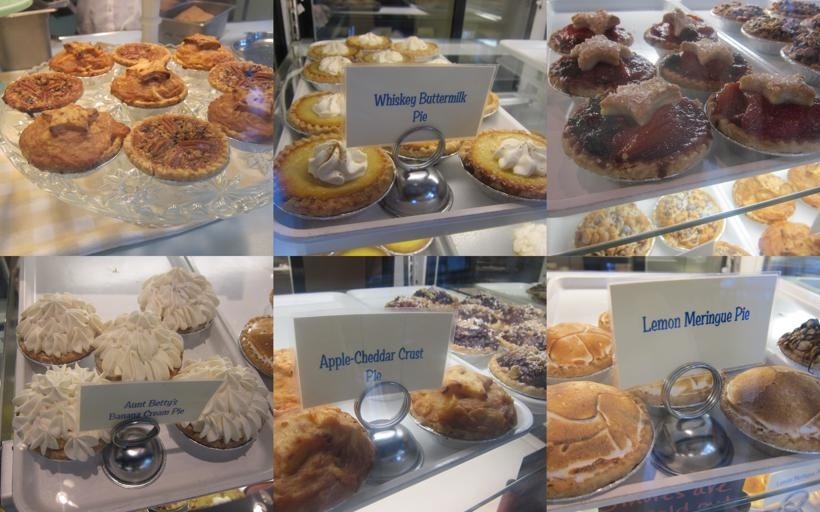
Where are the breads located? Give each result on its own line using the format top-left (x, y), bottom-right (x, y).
top-left (462, 292), bottom-right (509, 313)
top-left (48, 42), bottom-right (115, 78)
top-left (272, 132), bottom-right (399, 223)
top-left (788, 164), bottom-right (820, 209)
top-left (801, 12), bottom-right (820, 33)
top-left (704, 73), bottom-right (820, 160)
top-left (495, 303), bottom-right (544, 323)
top-left (285, 90), bottom-right (346, 133)
top-left (12, 364), bottom-right (124, 463)
top-left (112, 42), bottom-right (172, 67)
top-left (414, 284), bottom-right (458, 307)
top-left (188, 486), bottom-right (245, 512)
top-left (173, 33), bottom-right (236, 71)
top-left (764, 1), bottom-right (820, 24)
top-left (548, 10), bottom-right (635, 55)
top-left (458, 128), bottom-right (548, 207)
top-left (2, 73), bottom-right (85, 115)
top-left (642, 8), bottom-right (718, 57)
top-left (393, 35), bottom-right (439, 62)
top-left (270, 408), bottom-right (374, 510)
top-left (709, 0), bottom-right (763, 31)
top-left (779, 32), bottom-right (820, 86)
top-left (497, 318), bottom-right (547, 350)
top-left (302, 54), bottom-right (352, 88)
top-left (410, 365), bottom-right (517, 439)
top-left (720, 367), bottom-right (820, 458)
top-left (208, 61), bottom-right (274, 94)
top-left (547, 33), bottom-right (658, 99)
top-left (491, 344), bottom-right (546, 398)
top-left (654, 190), bottom-right (726, 251)
top-left (306, 41), bottom-right (355, 60)
top-left (546, 321), bottom-right (617, 386)
top-left (599, 310), bottom-right (615, 332)
top-left (547, 381), bottom-right (656, 501)
top-left (168, 358), bottom-right (274, 448)
top-left (384, 238), bottom-right (433, 257)
top-left (481, 90), bottom-right (499, 118)
top-left (329, 245), bottom-right (389, 258)
top-left (777, 317), bottom-right (820, 369)
top-left (360, 48), bottom-right (411, 66)
top-left (759, 221), bottom-right (820, 258)
top-left (451, 317), bottom-right (498, 355)
top-left (141, 267), bottom-right (220, 337)
top-left (95, 312), bottom-right (184, 384)
top-left (208, 88), bottom-right (274, 145)
top-left (19, 103), bottom-right (130, 175)
top-left (275, 350), bottom-right (303, 413)
top-left (456, 304), bottom-right (500, 328)
top-left (16, 292), bottom-right (103, 365)
top-left (660, 38), bottom-right (750, 92)
top-left (714, 241), bottom-right (752, 257)
top-left (125, 113), bottom-right (231, 182)
top-left (386, 297), bottom-right (424, 310)
top-left (613, 364), bottom-right (725, 417)
top-left (740, 15), bottom-right (810, 53)
top-left (240, 316), bottom-right (276, 377)
top-left (572, 204), bottom-right (655, 258)
top-left (384, 136), bottom-right (465, 163)
top-left (347, 31), bottom-right (393, 49)
top-left (112, 58), bottom-right (189, 108)
top-left (732, 174), bottom-right (796, 223)
top-left (561, 77), bottom-right (714, 184)
